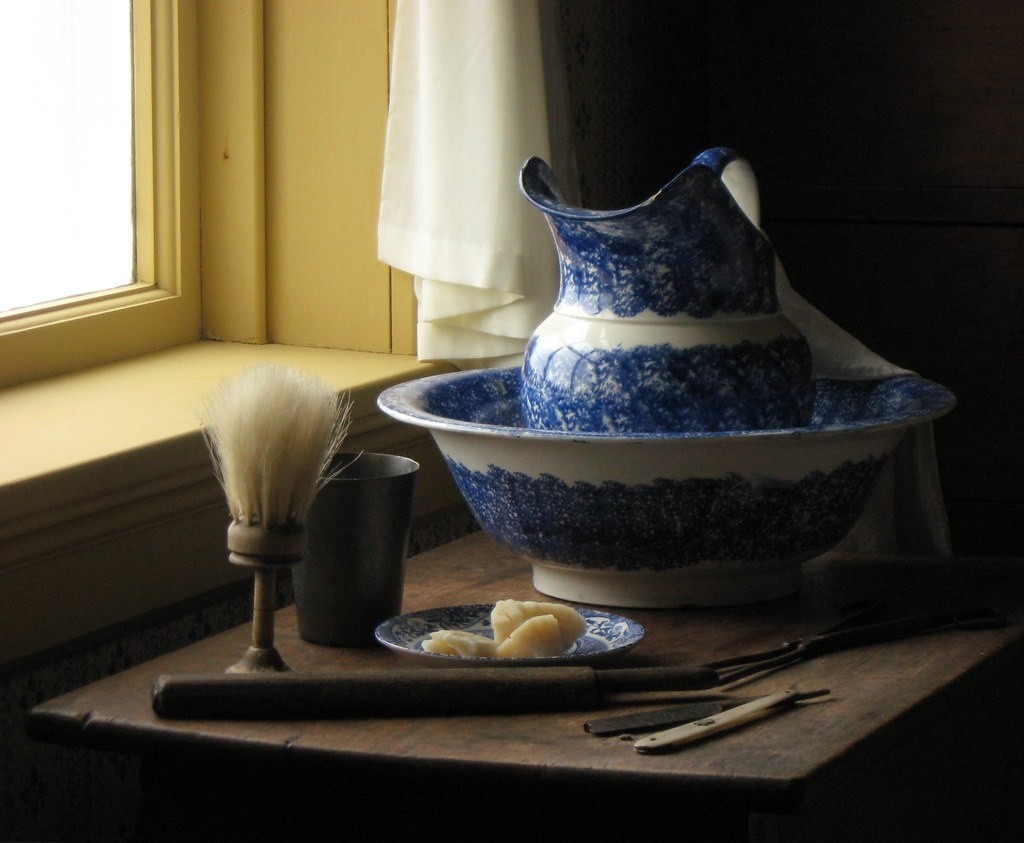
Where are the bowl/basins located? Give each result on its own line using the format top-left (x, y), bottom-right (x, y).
top-left (376, 367), bottom-right (954, 608)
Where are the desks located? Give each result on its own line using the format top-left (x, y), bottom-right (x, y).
top-left (22, 530), bottom-right (1024, 843)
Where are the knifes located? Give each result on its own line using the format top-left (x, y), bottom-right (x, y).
top-left (585, 687), bottom-right (831, 755)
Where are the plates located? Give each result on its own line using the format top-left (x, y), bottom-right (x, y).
top-left (375, 604), bottom-right (646, 669)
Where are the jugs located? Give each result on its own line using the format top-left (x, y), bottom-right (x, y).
top-left (515, 147), bottom-right (808, 433)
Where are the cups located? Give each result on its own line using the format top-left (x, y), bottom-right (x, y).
top-left (290, 451), bottom-right (419, 645)
